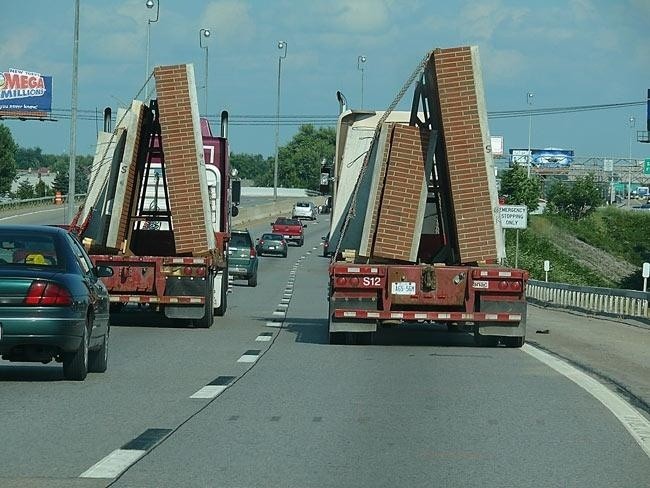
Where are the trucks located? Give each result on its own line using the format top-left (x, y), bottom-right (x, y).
top-left (60, 104), bottom-right (243, 329)
top-left (317, 88), bottom-right (531, 351)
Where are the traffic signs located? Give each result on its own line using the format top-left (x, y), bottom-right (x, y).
top-left (493, 201), bottom-right (529, 231)
top-left (643, 158), bottom-right (650, 174)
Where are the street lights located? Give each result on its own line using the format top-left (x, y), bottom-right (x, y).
top-left (141, 1), bottom-right (160, 103)
top-left (199, 25), bottom-right (211, 121)
top-left (623, 115), bottom-right (635, 210)
top-left (272, 41), bottom-right (287, 201)
top-left (524, 89), bottom-right (537, 181)
top-left (356, 53), bottom-right (367, 112)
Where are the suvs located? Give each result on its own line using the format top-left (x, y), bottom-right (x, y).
top-left (226, 226), bottom-right (261, 288)
top-left (271, 217), bottom-right (308, 247)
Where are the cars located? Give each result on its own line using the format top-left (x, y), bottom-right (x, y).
top-left (292, 202), bottom-right (319, 220)
top-left (624, 190), bottom-right (639, 200)
top-left (321, 231), bottom-right (334, 259)
top-left (0, 222), bottom-right (115, 383)
top-left (253, 231), bottom-right (289, 258)
